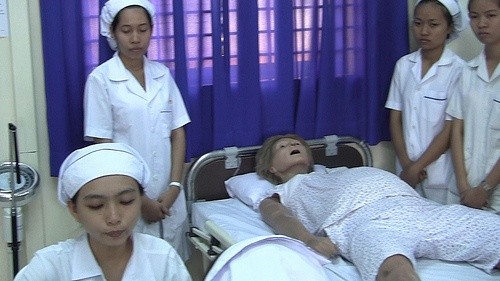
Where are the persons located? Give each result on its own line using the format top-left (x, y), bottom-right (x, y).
top-left (256, 135), bottom-right (500, 281)
top-left (444, 0), bottom-right (500, 214)
top-left (13, 142), bottom-right (192, 281)
top-left (384, 0), bottom-right (469, 206)
top-left (83, 0), bottom-right (191, 266)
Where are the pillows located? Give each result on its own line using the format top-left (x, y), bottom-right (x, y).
top-left (225, 164), bottom-right (324, 206)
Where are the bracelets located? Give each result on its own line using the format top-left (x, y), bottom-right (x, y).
top-left (169, 182), bottom-right (183, 190)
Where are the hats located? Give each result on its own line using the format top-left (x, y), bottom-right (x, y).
top-left (57, 143), bottom-right (152, 209)
top-left (414, 0), bottom-right (468, 45)
top-left (204, 234), bottom-right (327, 281)
top-left (100, 0), bottom-right (156, 50)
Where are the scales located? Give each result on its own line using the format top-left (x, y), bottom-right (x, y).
top-left (0, 123), bottom-right (40, 208)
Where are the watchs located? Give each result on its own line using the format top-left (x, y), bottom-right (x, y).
top-left (481, 180), bottom-right (494, 195)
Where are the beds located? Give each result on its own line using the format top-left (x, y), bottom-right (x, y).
top-left (186, 135), bottom-right (500, 281)
